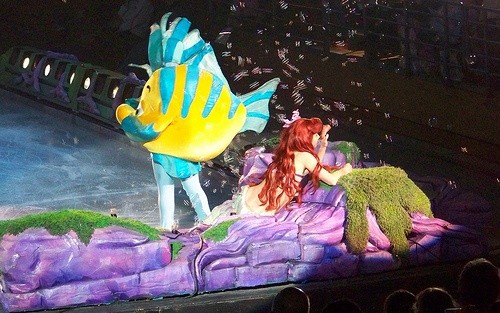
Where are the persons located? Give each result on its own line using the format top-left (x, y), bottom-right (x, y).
top-left (269, 255), bottom-right (500, 313)
top-left (208, 116), bottom-right (354, 230)
top-left (116, 63), bottom-right (220, 228)
